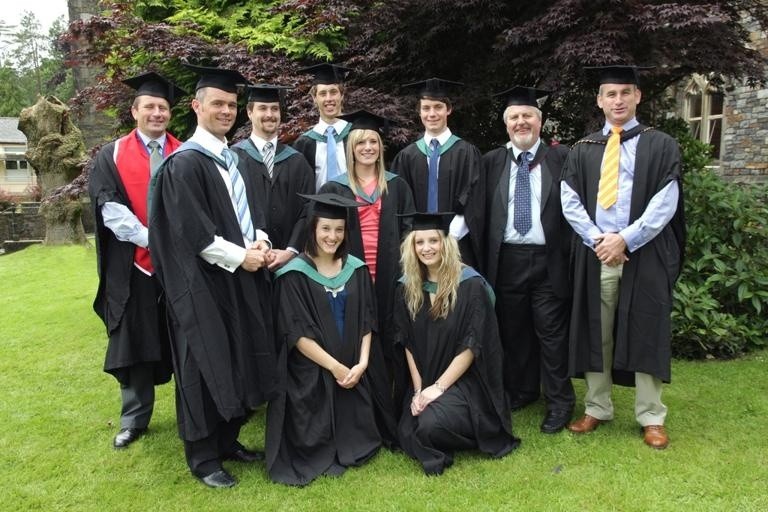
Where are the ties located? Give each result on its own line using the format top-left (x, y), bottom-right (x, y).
top-left (221, 149), bottom-right (255, 241)
top-left (513, 151), bottom-right (532, 236)
top-left (596, 126), bottom-right (624, 210)
top-left (262, 142), bottom-right (274, 179)
top-left (427, 138), bottom-right (439, 215)
top-left (147, 141), bottom-right (165, 178)
top-left (326, 126), bottom-right (338, 182)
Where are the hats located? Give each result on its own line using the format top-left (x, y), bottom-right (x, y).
top-left (582, 66), bottom-right (657, 85)
top-left (120, 70), bottom-right (190, 109)
top-left (492, 85), bottom-right (552, 109)
top-left (180, 63), bottom-right (254, 94)
top-left (334, 109), bottom-right (398, 132)
top-left (295, 192), bottom-right (371, 220)
top-left (296, 62), bottom-right (356, 85)
top-left (395, 212), bottom-right (456, 231)
top-left (402, 77), bottom-right (465, 98)
top-left (236, 83), bottom-right (296, 103)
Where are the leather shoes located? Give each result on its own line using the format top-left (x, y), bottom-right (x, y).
top-left (510, 399), bottom-right (528, 409)
top-left (539, 410), bottom-right (574, 434)
top-left (223, 447), bottom-right (265, 462)
top-left (200, 469), bottom-right (238, 488)
top-left (113, 426), bottom-right (148, 447)
top-left (567, 413), bottom-right (609, 432)
top-left (641, 425), bottom-right (669, 449)
